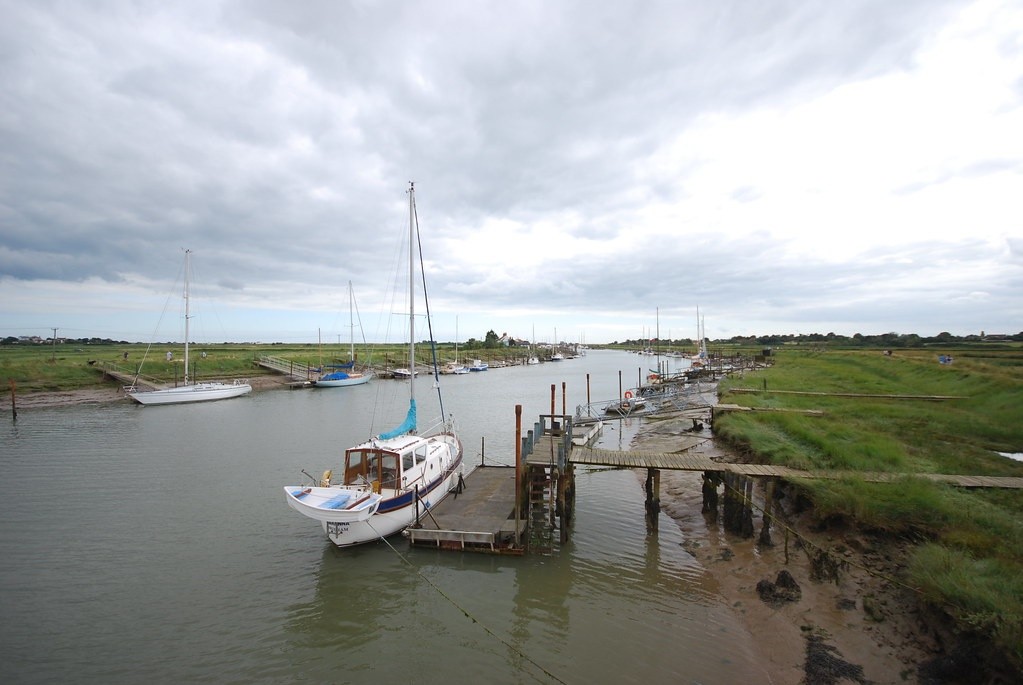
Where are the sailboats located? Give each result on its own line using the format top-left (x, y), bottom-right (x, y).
top-left (282, 178), bottom-right (465, 550)
top-left (527, 321), bottom-right (588, 364)
top-left (626, 324), bottom-right (656, 356)
top-left (121, 246), bottom-right (252, 405)
top-left (308, 278), bottom-right (375, 387)
top-left (665, 329), bottom-right (685, 358)
top-left (691, 314), bottom-right (710, 367)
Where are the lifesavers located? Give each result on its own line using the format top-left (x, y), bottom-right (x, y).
top-left (625, 391), bottom-right (632, 398)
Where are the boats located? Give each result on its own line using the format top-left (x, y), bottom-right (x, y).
top-left (605, 390), bottom-right (646, 413)
top-left (391, 355), bottom-right (489, 379)
top-left (645, 366), bottom-right (664, 384)
